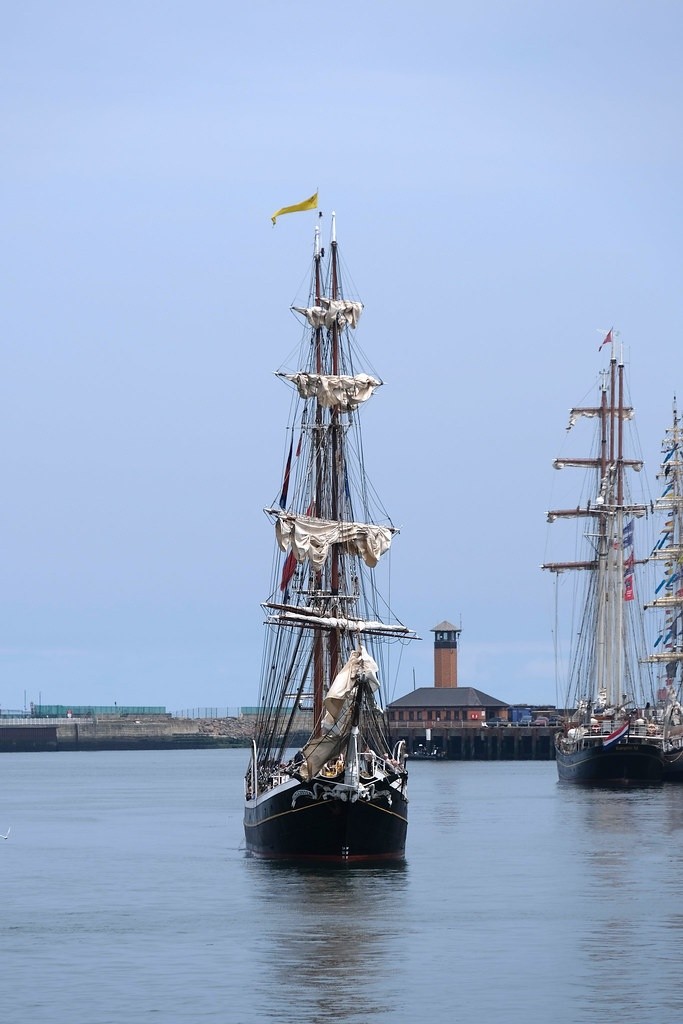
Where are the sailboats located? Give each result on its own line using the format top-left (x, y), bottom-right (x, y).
top-left (243, 190), bottom-right (421, 871)
top-left (544, 329), bottom-right (683, 796)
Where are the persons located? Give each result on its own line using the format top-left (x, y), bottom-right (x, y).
top-left (364, 747), bottom-right (377, 776)
top-left (294, 750), bottom-right (305, 774)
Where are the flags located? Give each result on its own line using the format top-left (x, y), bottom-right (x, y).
top-left (271, 191), bottom-right (319, 227)
top-left (598, 328), bottom-right (611, 353)
top-left (601, 719), bottom-right (630, 751)
top-left (623, 551), bottom-right (635, 601)
top-left (612, 519), bottom-right (632, 551)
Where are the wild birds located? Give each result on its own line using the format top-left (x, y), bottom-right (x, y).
top-left (0, 827), bottom-right (11, 840)
